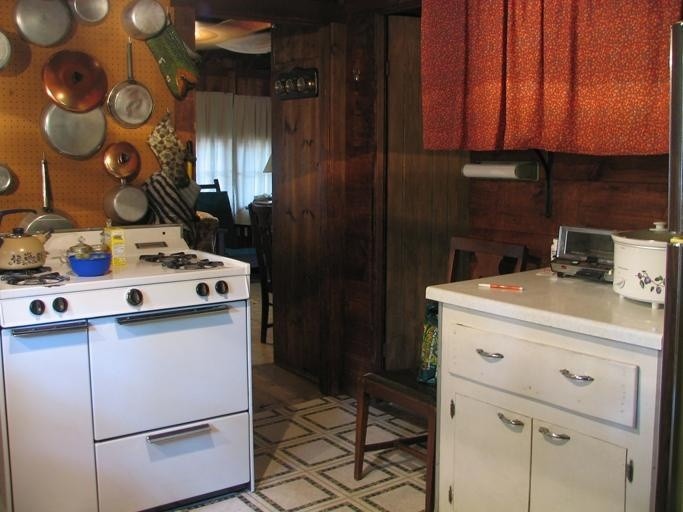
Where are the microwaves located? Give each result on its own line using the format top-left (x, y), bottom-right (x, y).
top-left (90, 414), bottom-right (252, 512)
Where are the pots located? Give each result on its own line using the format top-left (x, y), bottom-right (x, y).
top-left (0, 1), bottom-right (166, 231)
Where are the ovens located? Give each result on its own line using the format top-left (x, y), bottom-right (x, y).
top-left (87, 302), bottom-right (249, 441)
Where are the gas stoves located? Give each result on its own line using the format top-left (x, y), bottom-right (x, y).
top-left (0, 249), bottom-right (248, 298)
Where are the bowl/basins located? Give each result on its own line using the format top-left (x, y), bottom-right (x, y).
top-left (71, 251), bottom-right (112, 276)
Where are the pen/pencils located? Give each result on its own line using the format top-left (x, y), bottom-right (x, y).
top-left (477, 284), bottom-right (524, 291)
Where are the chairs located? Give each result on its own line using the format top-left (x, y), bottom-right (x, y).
top-left (247, 203), bottom-right (274, 345)
top-left (353, 236), bottom-right (530, 512)
top-left (194, 179), bottom-right (259, 269)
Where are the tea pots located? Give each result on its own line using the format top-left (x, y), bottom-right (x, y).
top-left (0, 208), bottom-right (55, 267)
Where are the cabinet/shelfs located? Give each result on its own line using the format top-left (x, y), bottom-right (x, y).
top-left (425, 266), bottom-right (666, 511)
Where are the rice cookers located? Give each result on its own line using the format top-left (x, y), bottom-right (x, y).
top-left (612, 220), bottom-right (668, 310)
